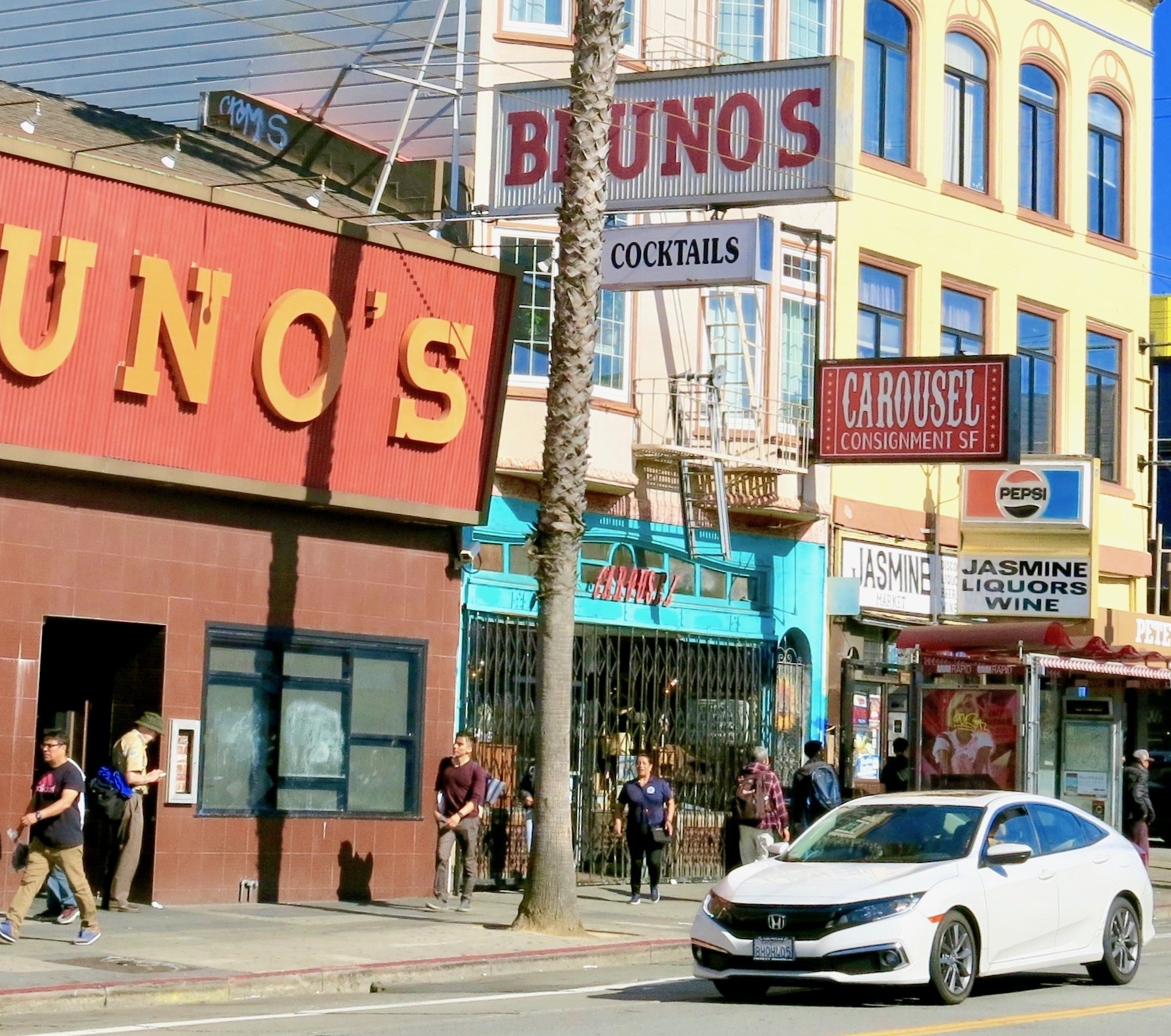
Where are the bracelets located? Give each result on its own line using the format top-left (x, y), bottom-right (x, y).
top-left (36, 810), bottom-right (42, 822)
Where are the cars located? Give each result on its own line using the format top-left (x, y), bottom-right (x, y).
top-left (685, 787), bottom-right (1158, 1006)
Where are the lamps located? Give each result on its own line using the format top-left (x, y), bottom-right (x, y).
top-left (71, 133), bottom-right (182, 170)
top-left (206, 175), bottom-right (327, 210)
top-left (339, 210), bottom-right (447, 241)
top-left (457, 244), bottom-right (553, 273)
top-left (0, 98), bottom-right (42, 135)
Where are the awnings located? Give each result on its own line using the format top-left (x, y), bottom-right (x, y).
top-left (898, 620), bottom-right (1170, 698)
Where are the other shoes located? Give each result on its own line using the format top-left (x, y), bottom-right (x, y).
top-left (427, 899), bottom-right (448, 910)
top-left (33, 906), bottom-right (64, 922)
top-left (456, 901), bottom-right (473, 911)
top-left (0, 920), bottom-right (16, 942)
top-left (57, 907), bottom-right (80, 923)
top-left (650, 888), bottom-right (660, 904)
top-left (72, 929), bottom-right (101, 946)
top-left (629, 895), bottom-right (641, 905)
top-left (109, 902), bottom-right (142, 913)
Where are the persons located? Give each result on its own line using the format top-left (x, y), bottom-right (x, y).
top-left (792, 740), bottom-right (842, 835)
top-left (34, 755), bottom-right (87, 926)
top-left (425, 731), bottom-right (486, 913)
top-left (1124, 749), bottom-right (1155, 867)
top-left (0, 728), bottom-right (102, 945)
top-left (514, 732), bottom-right (535, 893)
top-left (103, 711), bottom-right (165, 913)
top-left (737, 746), bottom-right (791, 865)
top-left (879, 738), bottom-right (910, 793)
top-left (932, 691), bottom-right (994, 792)
top-left (612, 753), bottom-right (675, 905)
top-left (964, 814), bottom-right (1013, 857)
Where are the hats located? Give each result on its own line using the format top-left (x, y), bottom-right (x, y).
top-left (134, 711), bottom-right (165, 736)
top-left (1132, 749), bottom-right (1154, 761)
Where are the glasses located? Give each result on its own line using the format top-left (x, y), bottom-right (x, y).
top-left (40, 743), bottom-right (66, 750)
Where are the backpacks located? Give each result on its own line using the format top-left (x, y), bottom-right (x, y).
top-left (809, 764), bottom-right (840, 810)
top-left (730, 769), bottom-right (767, 821)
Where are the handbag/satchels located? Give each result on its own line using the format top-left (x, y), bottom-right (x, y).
top-left (87, 777), bottom-right (126, 822)
top-left (650, 826), bottom-right (672, 844)
